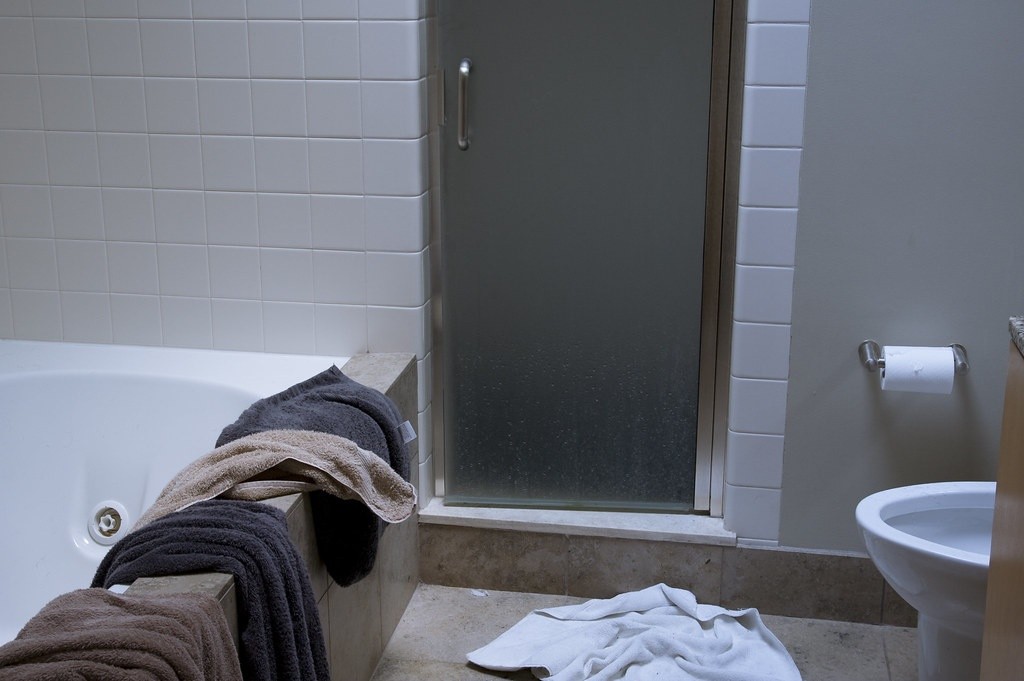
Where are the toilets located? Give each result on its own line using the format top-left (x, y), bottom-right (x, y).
top-left (854, 482), bottom-right (998, 681)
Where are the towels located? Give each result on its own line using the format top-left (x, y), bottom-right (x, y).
top-left (465, 583), bottom-right (803, 680)
top-left (215, 364), bottom-right (412, 588)
top-left (90, 500), bottom-right (331, 681)
top-left (0, 588), bottom-right (242, 680)
top-left (122, 430), bottom-right (417, 540)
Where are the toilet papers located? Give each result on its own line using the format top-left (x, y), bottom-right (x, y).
top-left (879, 346), bottom-right (954, 394)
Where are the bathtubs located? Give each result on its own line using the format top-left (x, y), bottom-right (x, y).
top-left (0, 340), bottom-right (417, 678)
